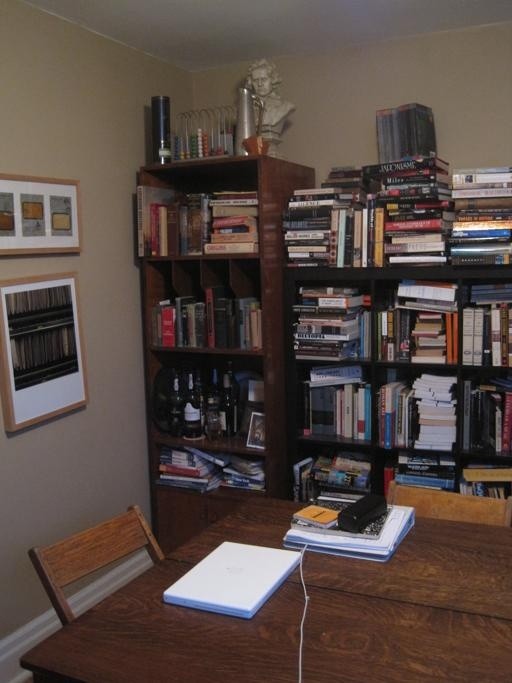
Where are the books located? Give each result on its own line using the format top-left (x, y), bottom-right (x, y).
top-left (281, 103), bottom-right (512, 268)
top-left (283, 503), bottom-right (416, 563)
top-left (150, 286), bottom-right (263, 352)
top-left (292, 279), bottom-right (512, 367)
top-left (136, 185), bottom-right (259, 257)
top-left (293, 450), bottom-right (512, 503)
top-left (156, 446), bottom-right (266, 491)
top-left (302, 365), bottom-right (512, 457)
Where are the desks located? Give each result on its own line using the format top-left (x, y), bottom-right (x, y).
top-left (19, 494), bottom-right (512, 683)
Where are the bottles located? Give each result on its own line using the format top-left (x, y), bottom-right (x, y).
top-left (168, 374), bottom-right (184, 436)
top-left (211, 367), bottom-right (220, 405)
top-left (183, 368), bottom-right (201, 438)
top-left (206, 393), bottom-right (222, 443)
top-left (152, 95), bottom-right (170, 164)
top-left (220, 372), bottom-right (237, 438)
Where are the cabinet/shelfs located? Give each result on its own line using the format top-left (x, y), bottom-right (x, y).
top-left (136, 154), bottom-right (318, 554)
top-left (290, 263), bottom-right (512, 512)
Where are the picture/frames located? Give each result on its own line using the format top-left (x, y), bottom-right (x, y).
top-left (2, 173), bottom-right (82, 256)
top-left (3, 271), bottom-right (89, 435)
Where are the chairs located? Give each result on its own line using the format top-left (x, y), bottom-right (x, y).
top-left (30, 505), bottom-right (165, 626)
top-left (388, 478), bottom-right (511, 528)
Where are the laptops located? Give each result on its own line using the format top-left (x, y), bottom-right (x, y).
top-left (162, 541), bottom-right (301, 620)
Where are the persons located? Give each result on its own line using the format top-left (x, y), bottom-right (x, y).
top-left (244, 56), bottom-right (297, 144)
